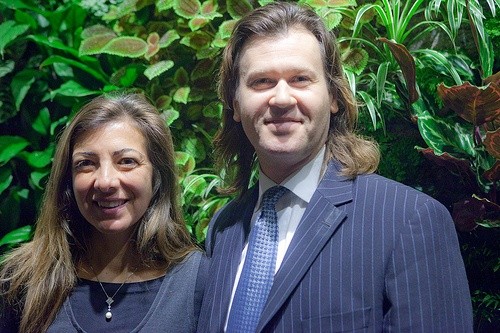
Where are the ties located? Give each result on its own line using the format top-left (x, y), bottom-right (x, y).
top-left (225, 184), bottom-right (293, 333)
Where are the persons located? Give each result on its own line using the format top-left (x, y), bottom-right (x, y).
top-left (0, 97), bottom-right (201, 333)
top-left (200, 3), bottom-right (474, 333)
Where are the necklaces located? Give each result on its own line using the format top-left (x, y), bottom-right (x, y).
top-left (85, 253), bottom-right (139, 319)
